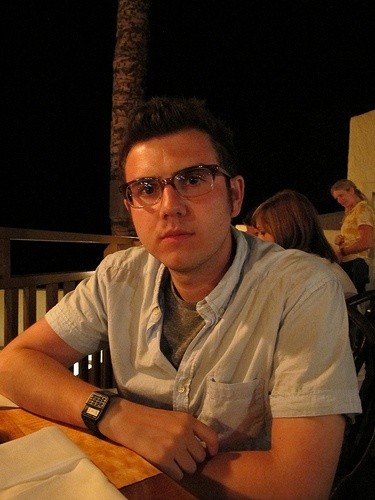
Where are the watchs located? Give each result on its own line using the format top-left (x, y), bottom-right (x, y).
top-left (81, 390), bottom-right (121, 438)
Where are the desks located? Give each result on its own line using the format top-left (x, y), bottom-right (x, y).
top-left (0, 392), bottom-right (197, 500)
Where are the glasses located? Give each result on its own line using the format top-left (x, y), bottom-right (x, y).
top-left (119, 164), bottom-right (231, 208)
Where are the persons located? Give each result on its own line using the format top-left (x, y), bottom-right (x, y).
top-left (0, 96), bottom-right (362, 500)
top-left (250, 180), bottom-right (374, 421)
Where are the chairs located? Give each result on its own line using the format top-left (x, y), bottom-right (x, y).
top-left (332, 290), bottom-right (375, 500)
